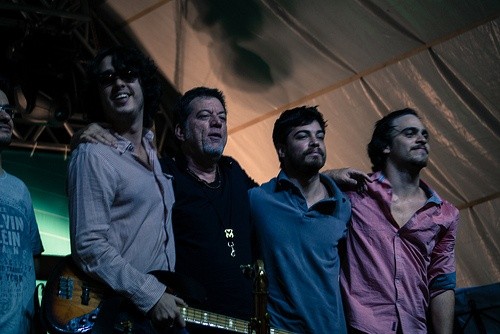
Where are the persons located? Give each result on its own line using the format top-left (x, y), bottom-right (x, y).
top-left (247, 104), bottom-right (352, 334)
top-left (0, 89), bottom-right (44, 334)
top-left (71, 86), bottom-right (373, 334)
top-left (65, 47), bottom-right (189, 334)
top-left (337, 107), bottom-right (460, 334)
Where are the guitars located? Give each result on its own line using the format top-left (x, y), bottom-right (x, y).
top-left (36, 253), bottom-right (294, 334)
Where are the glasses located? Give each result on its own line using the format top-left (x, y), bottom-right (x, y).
top-left (0, 105), bottom-right (13, 113)
top-left (99, 70), bottom-right (137, 84)
